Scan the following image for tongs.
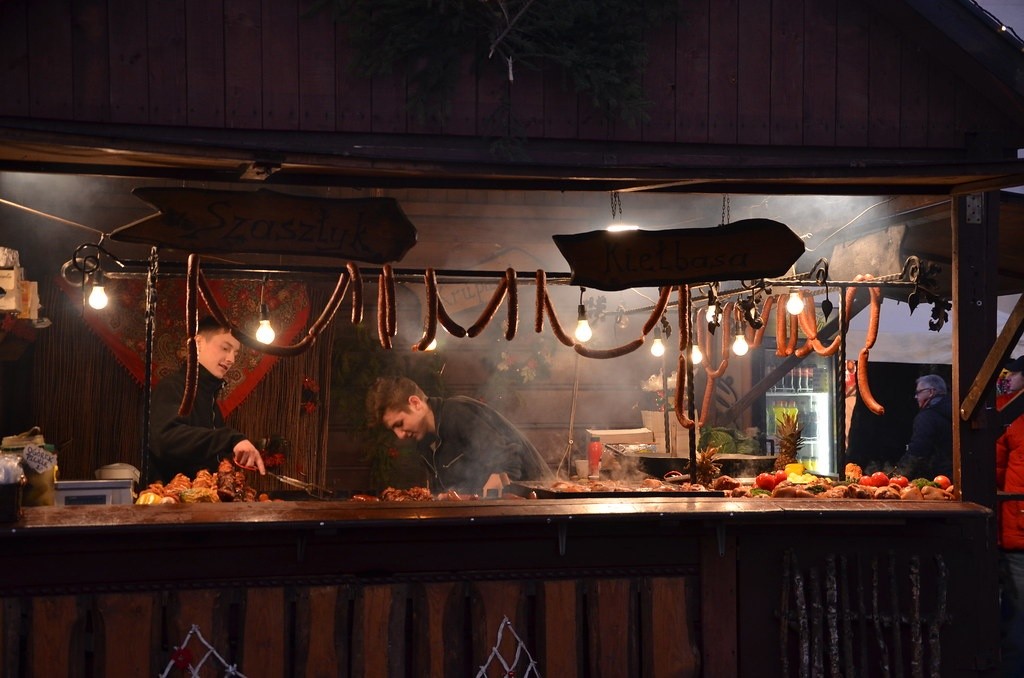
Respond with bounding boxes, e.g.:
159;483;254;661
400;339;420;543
233;455;333;501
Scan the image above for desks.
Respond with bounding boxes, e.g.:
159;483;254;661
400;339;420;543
1;497;994;678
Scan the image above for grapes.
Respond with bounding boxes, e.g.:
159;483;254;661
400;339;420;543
693;444;722;489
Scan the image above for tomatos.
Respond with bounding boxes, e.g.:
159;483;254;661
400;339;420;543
752;470;787;492
859;472;951;490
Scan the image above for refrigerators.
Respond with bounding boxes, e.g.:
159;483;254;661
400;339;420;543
734;336;845;482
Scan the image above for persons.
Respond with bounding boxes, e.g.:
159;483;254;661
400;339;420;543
146;315;266;490
993;357;1024;649
899;373;953;478
365;374;556;496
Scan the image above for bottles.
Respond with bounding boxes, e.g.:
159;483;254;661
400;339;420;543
768;359;817;393
588;437;602;476
766;400;817;437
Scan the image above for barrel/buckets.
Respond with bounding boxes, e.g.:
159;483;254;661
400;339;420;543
2;434;46;460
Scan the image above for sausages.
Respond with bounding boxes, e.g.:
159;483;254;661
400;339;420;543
178;254;883;428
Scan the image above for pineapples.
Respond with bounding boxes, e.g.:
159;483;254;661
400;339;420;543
775;413;806;471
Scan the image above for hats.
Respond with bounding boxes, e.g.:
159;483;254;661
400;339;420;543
1005;355;1024;371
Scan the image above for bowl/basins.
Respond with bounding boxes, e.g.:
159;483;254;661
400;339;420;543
575;460;601;479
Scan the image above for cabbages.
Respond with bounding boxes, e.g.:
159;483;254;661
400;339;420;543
696;427;761;455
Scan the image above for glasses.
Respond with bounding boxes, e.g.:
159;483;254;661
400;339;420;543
915;388;930;396
